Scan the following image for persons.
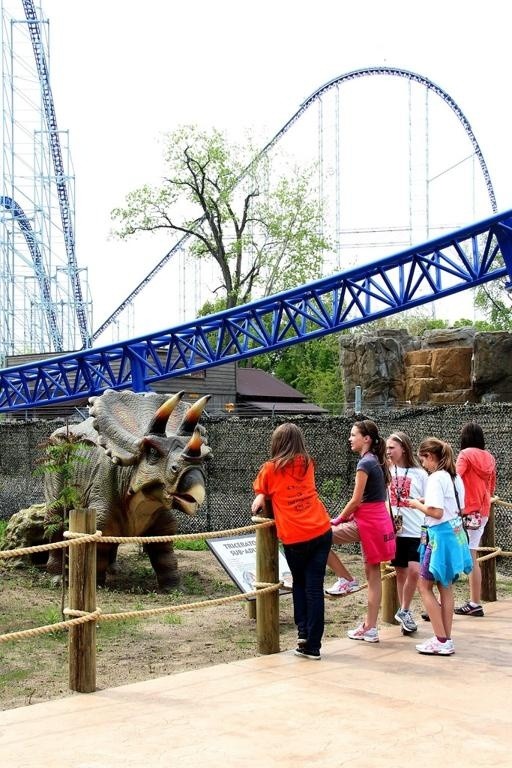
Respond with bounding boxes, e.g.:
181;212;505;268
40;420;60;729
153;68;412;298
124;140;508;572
253;424;333;660
456;423;497;618
404;436;474;656
325;420;395;643
386;433;428;635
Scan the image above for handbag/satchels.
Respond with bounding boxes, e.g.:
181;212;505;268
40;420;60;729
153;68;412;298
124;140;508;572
462;512;481;531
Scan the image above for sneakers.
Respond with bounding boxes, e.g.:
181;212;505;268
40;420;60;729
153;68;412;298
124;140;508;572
395;607;417;632
453;602;485;616
298;635;307;645
447;638;456;654
348;623;379;643
326;577;359;594
423;611;429;620
416;636;452;655
400;622;408;636
295;647;321;659
